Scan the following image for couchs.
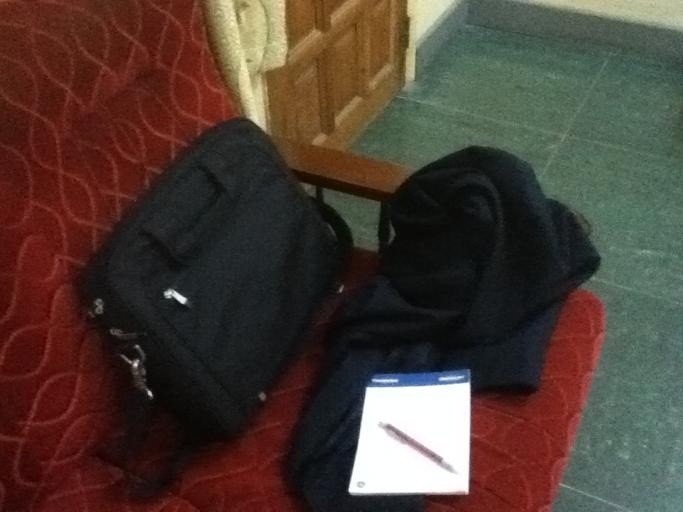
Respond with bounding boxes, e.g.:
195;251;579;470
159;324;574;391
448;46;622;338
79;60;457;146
0;0;604;512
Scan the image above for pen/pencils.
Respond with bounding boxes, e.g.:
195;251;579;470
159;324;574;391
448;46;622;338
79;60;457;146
378;420;457;474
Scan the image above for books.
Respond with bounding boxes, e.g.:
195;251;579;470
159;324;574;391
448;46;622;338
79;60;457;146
348;369;470;496
